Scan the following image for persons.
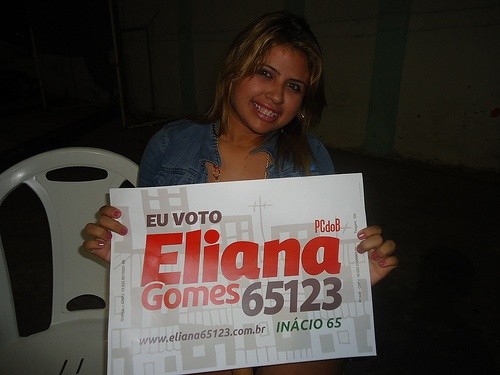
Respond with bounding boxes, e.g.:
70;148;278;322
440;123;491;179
81;11;400;375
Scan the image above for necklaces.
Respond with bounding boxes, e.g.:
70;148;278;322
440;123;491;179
211;134;269;179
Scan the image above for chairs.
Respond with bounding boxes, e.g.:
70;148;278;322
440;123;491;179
0;148;145;375
